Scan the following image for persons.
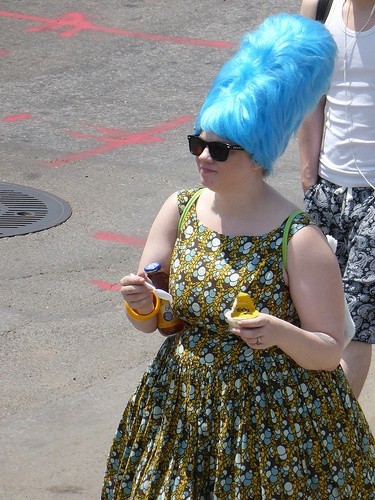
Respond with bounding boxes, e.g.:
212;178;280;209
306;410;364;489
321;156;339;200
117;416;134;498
100;13;375;500
297;0;375;399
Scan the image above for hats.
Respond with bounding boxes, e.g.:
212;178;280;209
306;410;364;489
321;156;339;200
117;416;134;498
195;13;337;176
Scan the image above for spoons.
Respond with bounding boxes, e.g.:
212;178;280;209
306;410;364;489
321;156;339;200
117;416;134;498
130;274;172;300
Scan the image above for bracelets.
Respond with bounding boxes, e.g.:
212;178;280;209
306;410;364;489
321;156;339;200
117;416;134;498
123;290;160;322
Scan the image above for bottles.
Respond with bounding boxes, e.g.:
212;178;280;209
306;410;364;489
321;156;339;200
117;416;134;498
144;262;185;336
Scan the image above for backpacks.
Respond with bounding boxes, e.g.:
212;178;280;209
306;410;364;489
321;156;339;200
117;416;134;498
178;188;356;351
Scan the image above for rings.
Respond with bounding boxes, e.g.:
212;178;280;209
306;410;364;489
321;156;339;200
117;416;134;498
257;337;261;344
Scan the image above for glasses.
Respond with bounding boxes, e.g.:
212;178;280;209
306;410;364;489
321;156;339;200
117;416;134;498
187;134;244;162
